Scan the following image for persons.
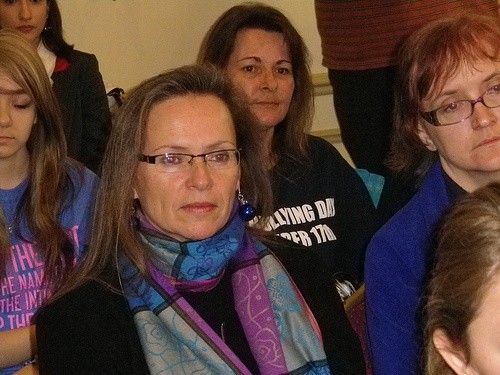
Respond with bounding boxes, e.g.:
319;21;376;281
0;0;113;179
0;26;101;375
196;1;385;301
364;12;500;375
315;0;500;221
35;64;367;375
423;181;500;375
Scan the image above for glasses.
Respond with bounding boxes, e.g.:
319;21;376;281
419;82;500;126
140;148;242;173
